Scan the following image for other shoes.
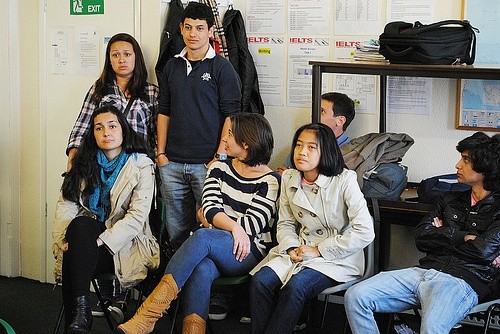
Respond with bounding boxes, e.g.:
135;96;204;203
107;302;127;323
240;295;251;325
208;292;229;320
91;298;111;316
293;303;314;331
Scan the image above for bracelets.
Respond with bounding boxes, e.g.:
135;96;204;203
156;152;166;158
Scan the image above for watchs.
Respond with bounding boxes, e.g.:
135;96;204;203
214;153;227;159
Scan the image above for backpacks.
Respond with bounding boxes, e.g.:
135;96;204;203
417;174;472;205
378;21;480;66
362;162;409;201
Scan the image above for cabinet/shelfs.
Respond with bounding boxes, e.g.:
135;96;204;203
309;58;500;271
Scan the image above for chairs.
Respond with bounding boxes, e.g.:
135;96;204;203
53;197;167;334
306;241;374;334
168;275;252;334
385;297;500;334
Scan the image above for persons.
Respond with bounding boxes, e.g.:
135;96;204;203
344;130;500;334
49;107;161;334
274;92;365;195
64;33;162;179
118;113;281;334
248;123;376;334
155;2;241;258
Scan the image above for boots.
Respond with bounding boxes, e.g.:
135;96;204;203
68;295;93;334
117;274;178;334
182;313;206;334
